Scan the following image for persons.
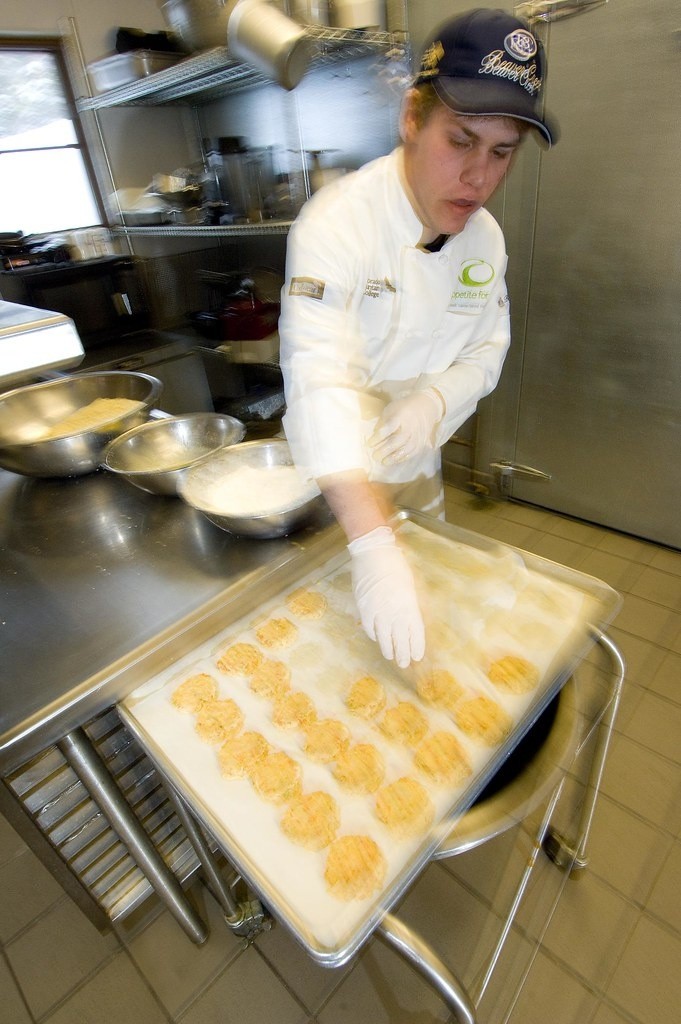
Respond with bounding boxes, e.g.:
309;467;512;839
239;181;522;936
278;8;561;670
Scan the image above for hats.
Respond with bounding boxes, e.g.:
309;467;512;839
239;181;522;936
413;7;557;150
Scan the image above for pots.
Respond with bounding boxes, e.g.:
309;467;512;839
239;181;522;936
184;279;279;341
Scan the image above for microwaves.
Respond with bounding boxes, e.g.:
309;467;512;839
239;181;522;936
1;254;159;353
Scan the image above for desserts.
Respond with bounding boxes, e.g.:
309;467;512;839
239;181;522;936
171;591;539;901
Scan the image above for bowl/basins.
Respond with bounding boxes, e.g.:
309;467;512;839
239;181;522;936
100;411;247;497
0;371;163;477
83;1;242;94
175;436;323;539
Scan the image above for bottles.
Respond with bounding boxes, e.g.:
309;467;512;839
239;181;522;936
196;132;311;223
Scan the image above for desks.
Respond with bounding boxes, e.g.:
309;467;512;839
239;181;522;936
0;408;343;952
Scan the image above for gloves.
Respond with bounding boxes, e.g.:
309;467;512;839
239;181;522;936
345;525;427;669
370;386;444;466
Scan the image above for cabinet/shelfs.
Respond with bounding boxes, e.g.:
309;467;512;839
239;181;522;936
57;0;413;376
